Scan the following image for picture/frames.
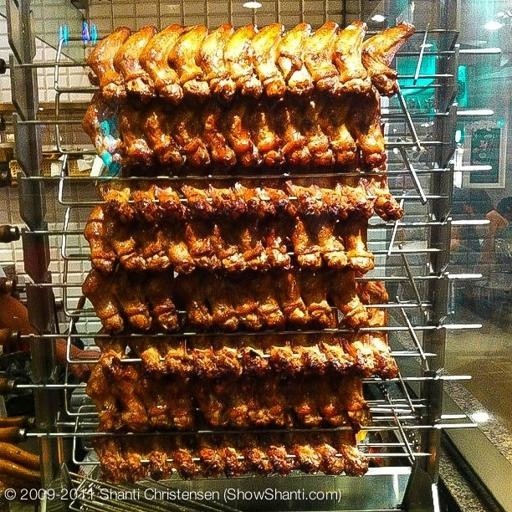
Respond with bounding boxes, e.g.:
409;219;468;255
460;121;507;189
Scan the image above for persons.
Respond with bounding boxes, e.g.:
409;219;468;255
2;271;101;381
451;185;511;279
